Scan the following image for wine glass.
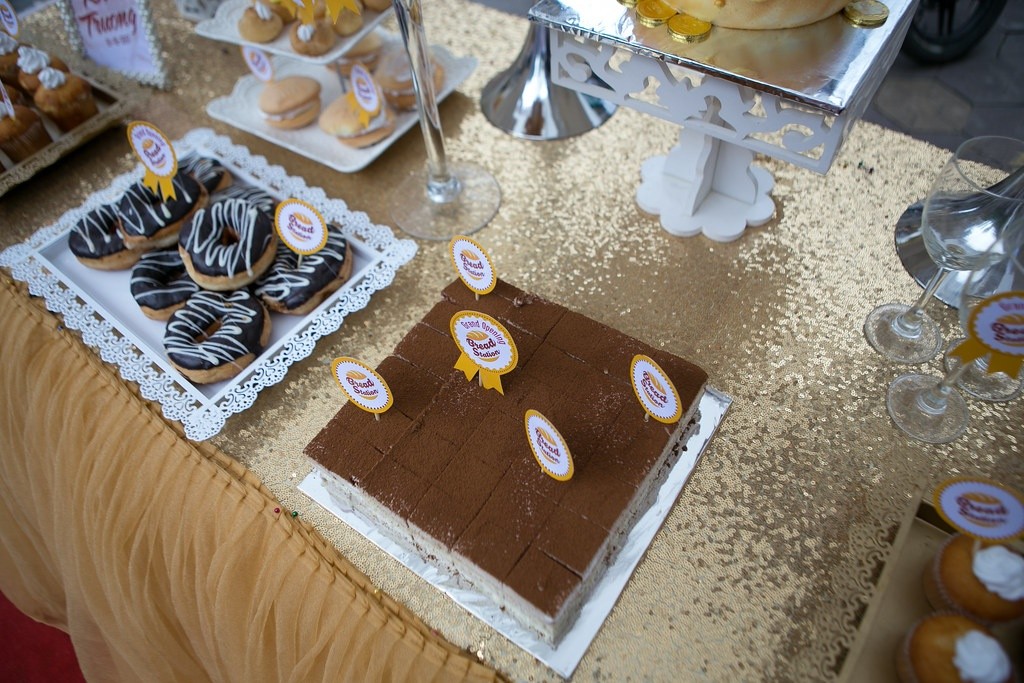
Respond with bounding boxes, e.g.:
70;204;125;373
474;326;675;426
886;218;1022;445
945;202;1023;400
390;1;502;242
866;136;1024;365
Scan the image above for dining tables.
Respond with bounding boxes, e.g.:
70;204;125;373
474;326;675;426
0;0;1024;682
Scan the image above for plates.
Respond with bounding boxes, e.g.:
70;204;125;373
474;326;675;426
1;68;134;205
0;125;418;446
204;32;477;175
193;1;395;65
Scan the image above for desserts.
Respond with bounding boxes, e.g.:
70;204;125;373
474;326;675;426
0;1;714;651
897;529;1024;682
